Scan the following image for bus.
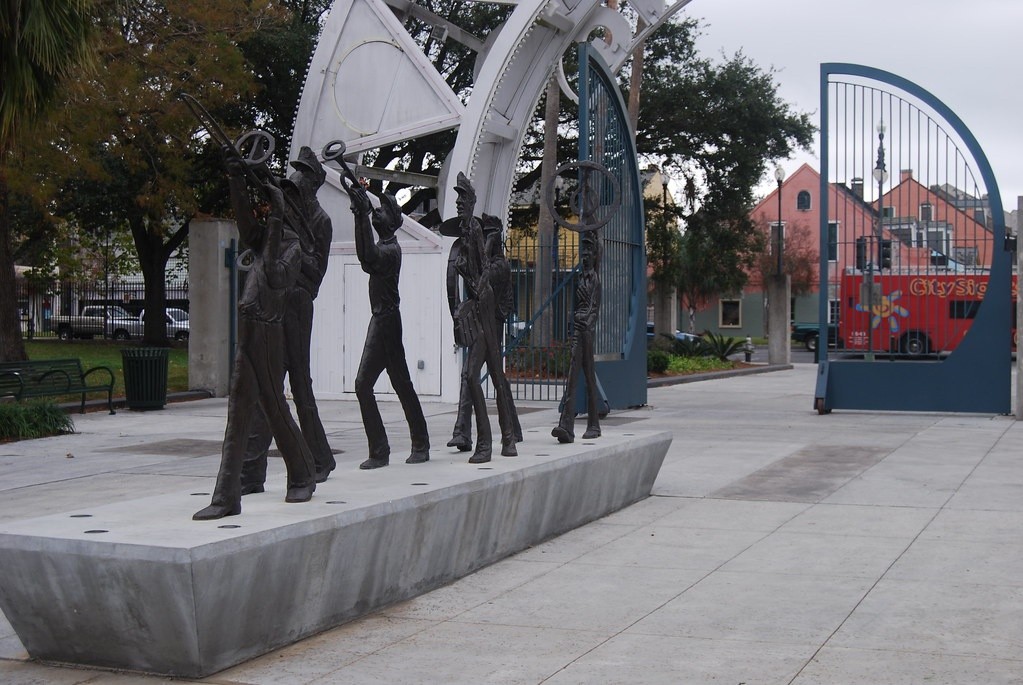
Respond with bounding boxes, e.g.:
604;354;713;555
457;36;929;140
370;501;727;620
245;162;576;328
839;266;1021;360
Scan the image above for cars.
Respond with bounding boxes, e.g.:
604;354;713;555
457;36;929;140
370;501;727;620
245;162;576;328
647;322;701;344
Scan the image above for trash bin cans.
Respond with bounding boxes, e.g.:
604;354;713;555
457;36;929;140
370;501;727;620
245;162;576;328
120;349;167;412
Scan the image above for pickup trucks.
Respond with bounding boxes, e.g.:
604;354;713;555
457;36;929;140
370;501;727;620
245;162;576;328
51;305;129;340
106;309;189;342
791;321;844;351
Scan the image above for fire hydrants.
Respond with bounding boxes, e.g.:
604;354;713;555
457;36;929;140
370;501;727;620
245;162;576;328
744;335;754;361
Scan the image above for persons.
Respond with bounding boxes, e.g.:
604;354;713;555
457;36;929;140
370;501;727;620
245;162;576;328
552;232;602;442
350;184;431;469
446;174;523;464
193;148;336;520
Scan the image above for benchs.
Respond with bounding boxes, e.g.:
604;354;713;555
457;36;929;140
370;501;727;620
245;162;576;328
0;357;117;414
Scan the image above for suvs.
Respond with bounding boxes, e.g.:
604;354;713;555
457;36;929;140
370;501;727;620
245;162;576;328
505;314;530;342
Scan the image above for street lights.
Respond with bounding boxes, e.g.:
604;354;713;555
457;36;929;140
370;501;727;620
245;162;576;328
874;120;888;267
775;164;784;274
